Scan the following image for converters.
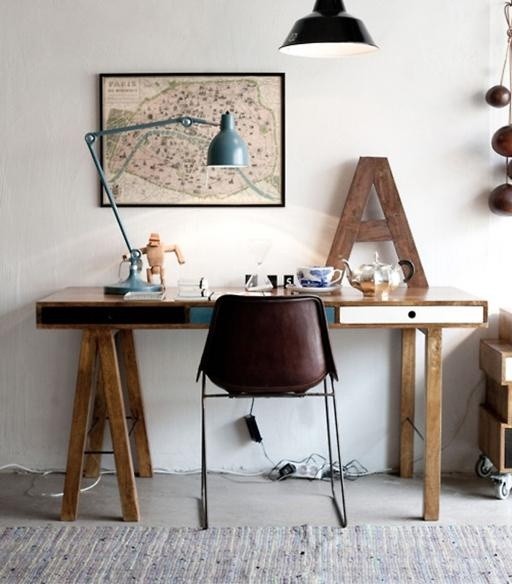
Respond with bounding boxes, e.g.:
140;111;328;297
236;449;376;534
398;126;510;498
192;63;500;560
243;410;262;446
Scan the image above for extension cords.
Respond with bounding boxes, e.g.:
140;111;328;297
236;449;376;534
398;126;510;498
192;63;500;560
282;462;323;480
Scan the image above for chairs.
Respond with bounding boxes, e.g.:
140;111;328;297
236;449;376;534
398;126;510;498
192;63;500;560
196;294;348;527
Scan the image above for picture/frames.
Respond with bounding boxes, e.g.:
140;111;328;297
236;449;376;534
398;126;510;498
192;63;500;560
97;73;285;208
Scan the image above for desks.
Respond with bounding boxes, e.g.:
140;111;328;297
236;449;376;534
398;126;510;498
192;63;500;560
37;285;488;522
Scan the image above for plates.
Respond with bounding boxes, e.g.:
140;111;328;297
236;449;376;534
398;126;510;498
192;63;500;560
285;284;342;294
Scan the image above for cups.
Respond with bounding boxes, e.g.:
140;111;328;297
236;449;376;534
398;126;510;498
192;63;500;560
297;266;344;287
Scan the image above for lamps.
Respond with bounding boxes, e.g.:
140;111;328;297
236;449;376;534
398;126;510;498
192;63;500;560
84;110;250;297
279;0;381;59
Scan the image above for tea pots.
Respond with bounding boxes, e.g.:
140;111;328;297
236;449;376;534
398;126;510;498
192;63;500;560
339;251;416;298
